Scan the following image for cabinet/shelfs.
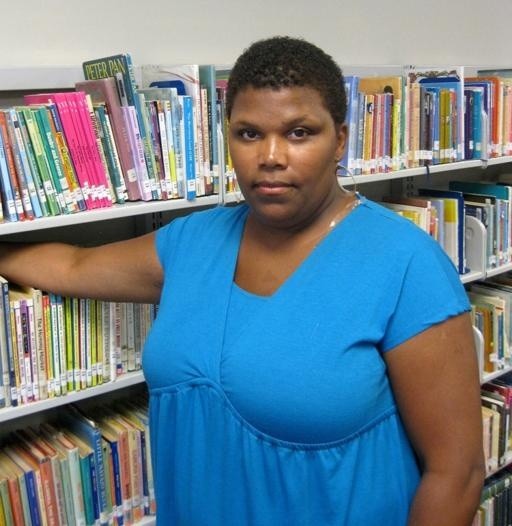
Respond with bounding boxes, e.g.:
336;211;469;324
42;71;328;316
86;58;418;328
0;153;512;525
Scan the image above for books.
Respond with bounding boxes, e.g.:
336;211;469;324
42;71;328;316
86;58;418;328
0;51;242;526
336;64;512;526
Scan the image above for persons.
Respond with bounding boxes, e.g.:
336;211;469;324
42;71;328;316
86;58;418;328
0;36;485;526
